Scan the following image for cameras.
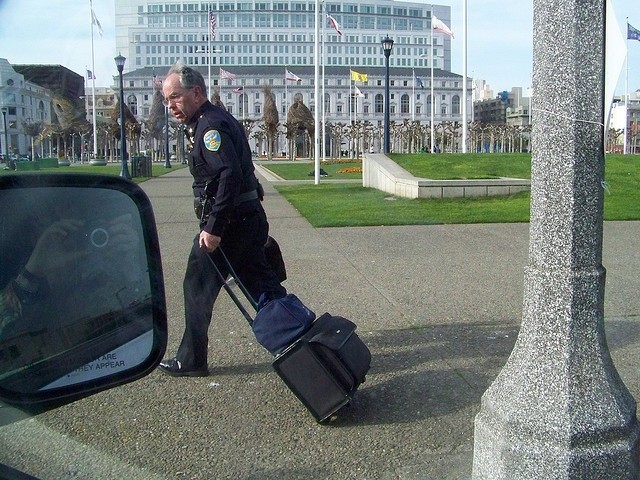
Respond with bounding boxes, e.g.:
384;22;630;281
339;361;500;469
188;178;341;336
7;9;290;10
77;212;123;259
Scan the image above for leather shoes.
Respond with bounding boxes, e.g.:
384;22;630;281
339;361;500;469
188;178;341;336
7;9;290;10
158;357;209;377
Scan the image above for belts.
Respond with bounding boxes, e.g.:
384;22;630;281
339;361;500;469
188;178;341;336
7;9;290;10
230;189;259;206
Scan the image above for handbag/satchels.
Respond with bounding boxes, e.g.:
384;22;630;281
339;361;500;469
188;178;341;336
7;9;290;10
252;291;316;356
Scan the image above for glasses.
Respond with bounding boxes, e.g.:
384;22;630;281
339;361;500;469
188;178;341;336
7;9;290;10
161;89;194;108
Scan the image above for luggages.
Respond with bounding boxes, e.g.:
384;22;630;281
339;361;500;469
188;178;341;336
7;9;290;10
200;239;371;424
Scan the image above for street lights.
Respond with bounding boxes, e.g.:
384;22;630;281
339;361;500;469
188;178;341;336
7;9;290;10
1;108;9;169
115;286;129;324
195;49;223;103
113;51;133;180
380;34;394;153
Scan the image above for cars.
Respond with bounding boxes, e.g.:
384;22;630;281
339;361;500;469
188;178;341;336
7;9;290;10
0;315;154;393
0;170;168;427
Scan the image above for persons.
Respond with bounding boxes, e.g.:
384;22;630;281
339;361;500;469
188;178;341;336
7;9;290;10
156;60;269;377
0;190;145;364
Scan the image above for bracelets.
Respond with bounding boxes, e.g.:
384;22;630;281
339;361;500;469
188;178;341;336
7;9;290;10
18;264;47;287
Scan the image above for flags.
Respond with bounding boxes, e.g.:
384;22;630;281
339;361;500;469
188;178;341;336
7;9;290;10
153;72;162;83
87;70;97;80
91;9;104;36
626;24;640;40
351;70;368;82
221;69;235;81
209;10;218;40
432;15;455;39
329;15;343;35
355;86;365;98
414;73;425;89
232;86;244;94
286;70;303;82
